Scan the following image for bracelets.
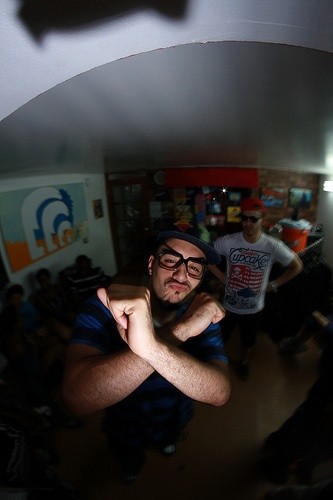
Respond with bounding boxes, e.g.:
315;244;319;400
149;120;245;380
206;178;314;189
271;281;277;293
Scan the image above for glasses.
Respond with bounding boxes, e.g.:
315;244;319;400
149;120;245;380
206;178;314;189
241;215;261;224
157;249;209;278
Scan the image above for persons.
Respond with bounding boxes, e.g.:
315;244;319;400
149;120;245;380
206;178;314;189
0;196;310;485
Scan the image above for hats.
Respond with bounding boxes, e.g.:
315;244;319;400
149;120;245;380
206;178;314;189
150;215;220;264
235;197;268;217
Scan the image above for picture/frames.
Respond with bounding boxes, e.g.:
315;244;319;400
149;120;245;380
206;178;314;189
259;186;285;209
287;187;312;209
